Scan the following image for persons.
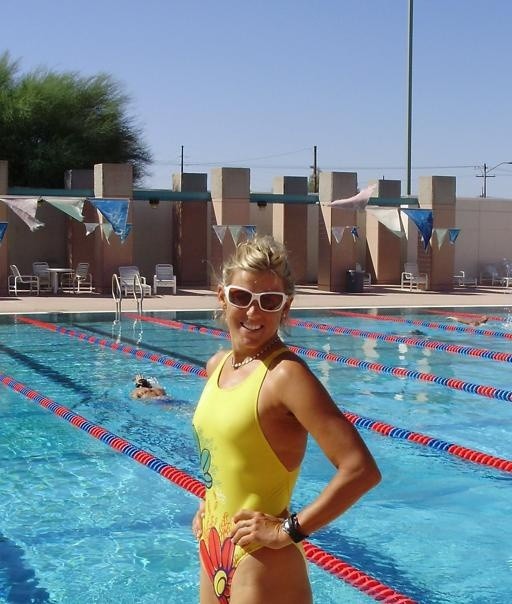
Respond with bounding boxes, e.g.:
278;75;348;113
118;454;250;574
191;227;383;603
130;370;196;415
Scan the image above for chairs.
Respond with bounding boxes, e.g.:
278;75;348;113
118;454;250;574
7;262;92;297
153;263;177;294
355;262;372;289
119;265;152;297
453;271;512;287
401;262;428;291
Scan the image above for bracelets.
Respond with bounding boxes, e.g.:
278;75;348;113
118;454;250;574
282;510;309;545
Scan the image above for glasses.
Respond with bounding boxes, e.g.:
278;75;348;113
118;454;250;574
224;285;288;312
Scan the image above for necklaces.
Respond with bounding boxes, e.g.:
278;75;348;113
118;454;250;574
230;334;281;368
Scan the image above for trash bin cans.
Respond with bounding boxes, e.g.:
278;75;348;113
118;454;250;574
347;270;364;293
48;256;66;268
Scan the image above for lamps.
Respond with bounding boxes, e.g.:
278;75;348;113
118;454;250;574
149;199;159;209
257;201;267;211
